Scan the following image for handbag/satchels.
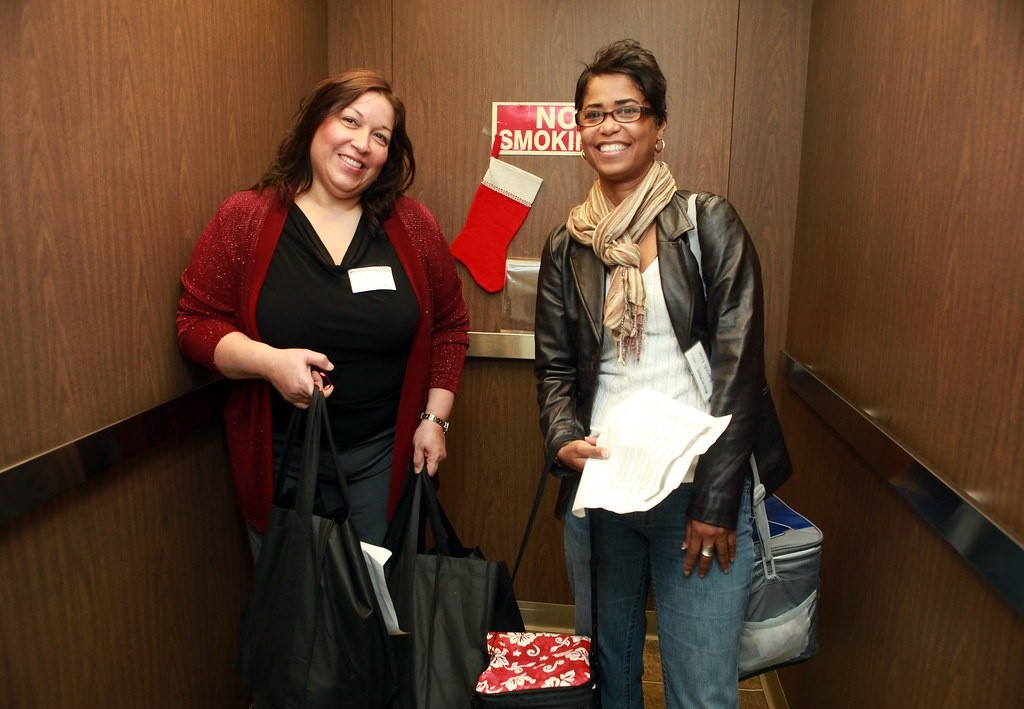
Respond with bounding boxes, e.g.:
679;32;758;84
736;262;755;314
234;385;401;709
380;457;526;709
475;628;598;709
738;492;824;681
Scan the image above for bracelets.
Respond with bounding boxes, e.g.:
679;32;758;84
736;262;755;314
419;411;451;435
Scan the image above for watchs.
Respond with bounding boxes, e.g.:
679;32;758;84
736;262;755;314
552;439;577;470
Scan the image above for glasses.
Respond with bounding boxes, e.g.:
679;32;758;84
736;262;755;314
575;104;654;128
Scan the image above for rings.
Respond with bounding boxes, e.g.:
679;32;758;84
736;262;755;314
701;545;714;558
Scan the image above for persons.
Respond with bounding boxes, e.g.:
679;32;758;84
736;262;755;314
533;37;791;709
178;69;469;708
